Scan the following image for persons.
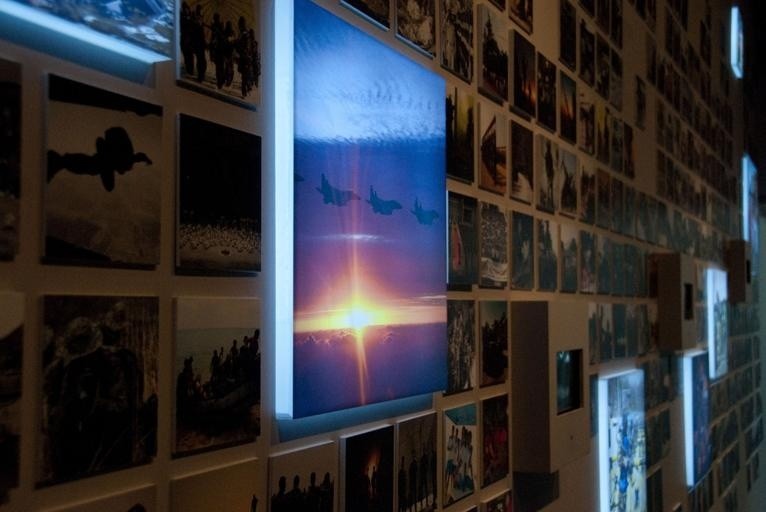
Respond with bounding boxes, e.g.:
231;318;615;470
443;425;477;497
269;469;332;511
179;327;261;417
180;4;261;99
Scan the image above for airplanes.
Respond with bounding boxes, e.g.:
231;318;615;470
311;171;441;228
